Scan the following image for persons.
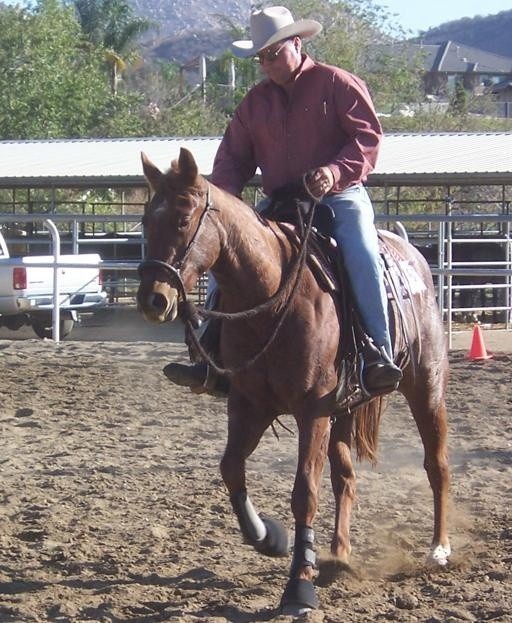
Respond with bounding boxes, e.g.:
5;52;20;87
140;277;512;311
162;6;403;398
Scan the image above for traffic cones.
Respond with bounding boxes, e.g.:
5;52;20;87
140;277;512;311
464;323;495;359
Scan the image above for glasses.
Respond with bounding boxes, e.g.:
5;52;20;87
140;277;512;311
251;38;293;66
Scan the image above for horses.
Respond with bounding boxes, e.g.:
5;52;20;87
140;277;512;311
135;146;452;617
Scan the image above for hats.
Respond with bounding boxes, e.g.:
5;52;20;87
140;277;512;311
230;5;323;57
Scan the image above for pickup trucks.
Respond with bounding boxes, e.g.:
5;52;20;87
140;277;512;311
0;228;109;341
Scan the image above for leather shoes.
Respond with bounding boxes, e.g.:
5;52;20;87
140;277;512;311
162;362;208;395
366;364;403;391
